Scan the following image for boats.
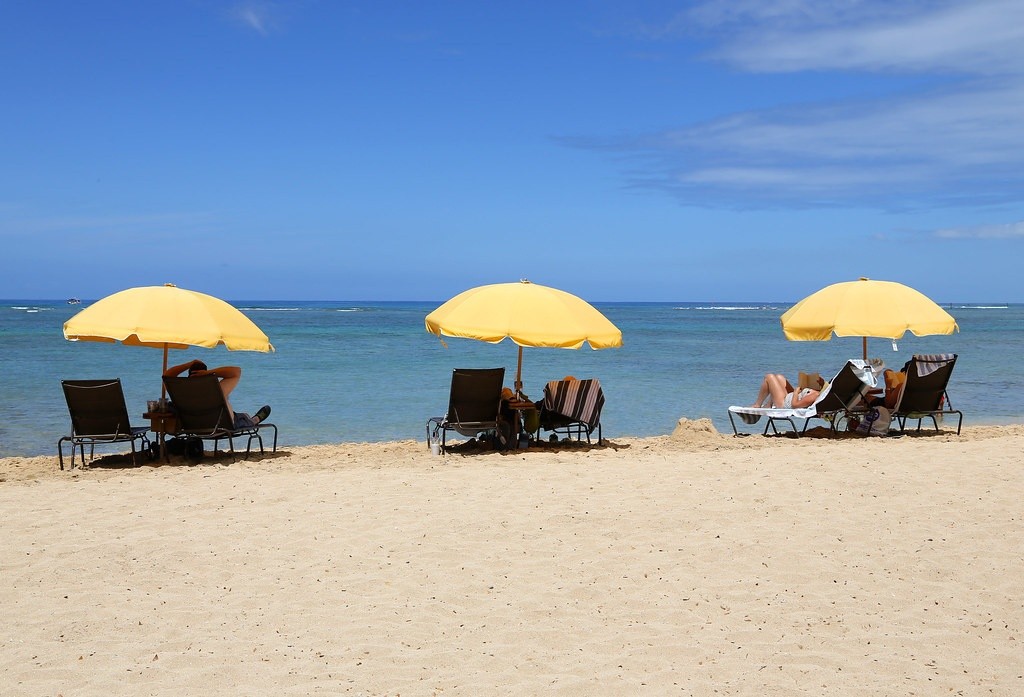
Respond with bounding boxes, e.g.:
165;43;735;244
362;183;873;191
67;299;80;305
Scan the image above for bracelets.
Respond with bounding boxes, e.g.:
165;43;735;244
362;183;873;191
885;388;891;391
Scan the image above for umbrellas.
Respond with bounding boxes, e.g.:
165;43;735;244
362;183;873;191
425;279;623;434
780;278;960;420
62;282;275;459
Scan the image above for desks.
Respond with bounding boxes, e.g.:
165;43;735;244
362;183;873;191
143;412;176;463
868;387;883;394
511;402;535;449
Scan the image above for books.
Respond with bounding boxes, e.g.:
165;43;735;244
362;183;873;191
798;372;820;391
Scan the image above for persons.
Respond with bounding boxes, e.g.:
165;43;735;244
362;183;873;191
535;375;576;410
855;360;911;408
163;358;271;430
749;374;834;408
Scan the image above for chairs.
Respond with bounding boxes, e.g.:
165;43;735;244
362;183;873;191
531;379;605;446
58;377;157;470
427;367;511;456
728;357;885;438
887;354;963;436
162;374;278;463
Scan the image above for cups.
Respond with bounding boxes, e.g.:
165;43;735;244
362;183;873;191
159;397;168;414
146;400;158;413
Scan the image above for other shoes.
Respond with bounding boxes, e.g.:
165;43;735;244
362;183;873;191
251;405;271;426
